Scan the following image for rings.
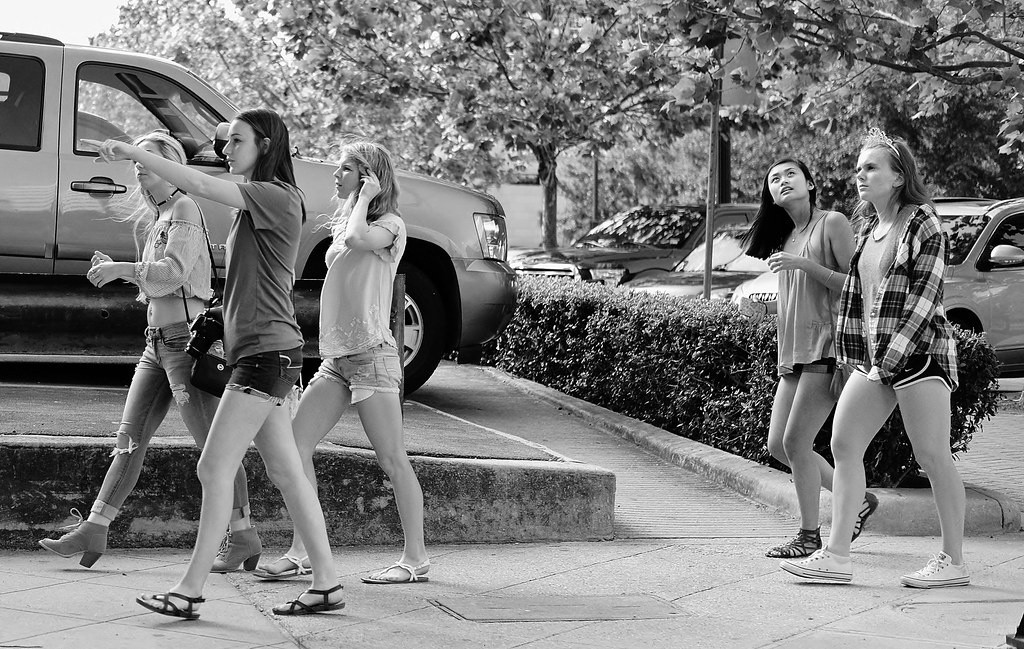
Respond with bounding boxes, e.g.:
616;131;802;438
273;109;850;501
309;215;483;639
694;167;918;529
92;274;97;279
90;268;96;273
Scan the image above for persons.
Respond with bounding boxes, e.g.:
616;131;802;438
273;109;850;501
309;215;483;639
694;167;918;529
253;143;433;585
778;127;972;588
80;109;347;620
40;131;262;573
737;157;878;559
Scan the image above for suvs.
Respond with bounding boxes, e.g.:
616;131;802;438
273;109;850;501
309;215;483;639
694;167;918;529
0;31;519;399
731;197;1024;408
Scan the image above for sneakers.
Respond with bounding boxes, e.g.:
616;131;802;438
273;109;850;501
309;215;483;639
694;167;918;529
781;546;853;582
900;551;972;588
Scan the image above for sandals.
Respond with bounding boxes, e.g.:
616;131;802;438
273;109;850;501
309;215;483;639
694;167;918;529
360;558;429;584
851;491;878;541
254;553;312;579
135;590;205;619
765;526;822;559
271;584;345;615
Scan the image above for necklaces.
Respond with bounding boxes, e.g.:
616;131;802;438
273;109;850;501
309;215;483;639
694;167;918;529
155;187;180;207
790;208;816;242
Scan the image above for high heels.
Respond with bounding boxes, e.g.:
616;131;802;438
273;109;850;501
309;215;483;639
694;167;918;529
208;525;262;573
38;508;108;568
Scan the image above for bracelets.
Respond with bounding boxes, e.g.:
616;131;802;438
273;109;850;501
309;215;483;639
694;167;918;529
824;270;834;286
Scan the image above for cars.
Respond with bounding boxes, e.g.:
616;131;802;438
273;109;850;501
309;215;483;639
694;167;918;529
506;202;760;289
615;221;783;302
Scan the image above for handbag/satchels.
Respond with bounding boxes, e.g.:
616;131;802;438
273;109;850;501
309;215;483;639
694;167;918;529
187;353;230;398
185;303;225;359
286;384;303;420
